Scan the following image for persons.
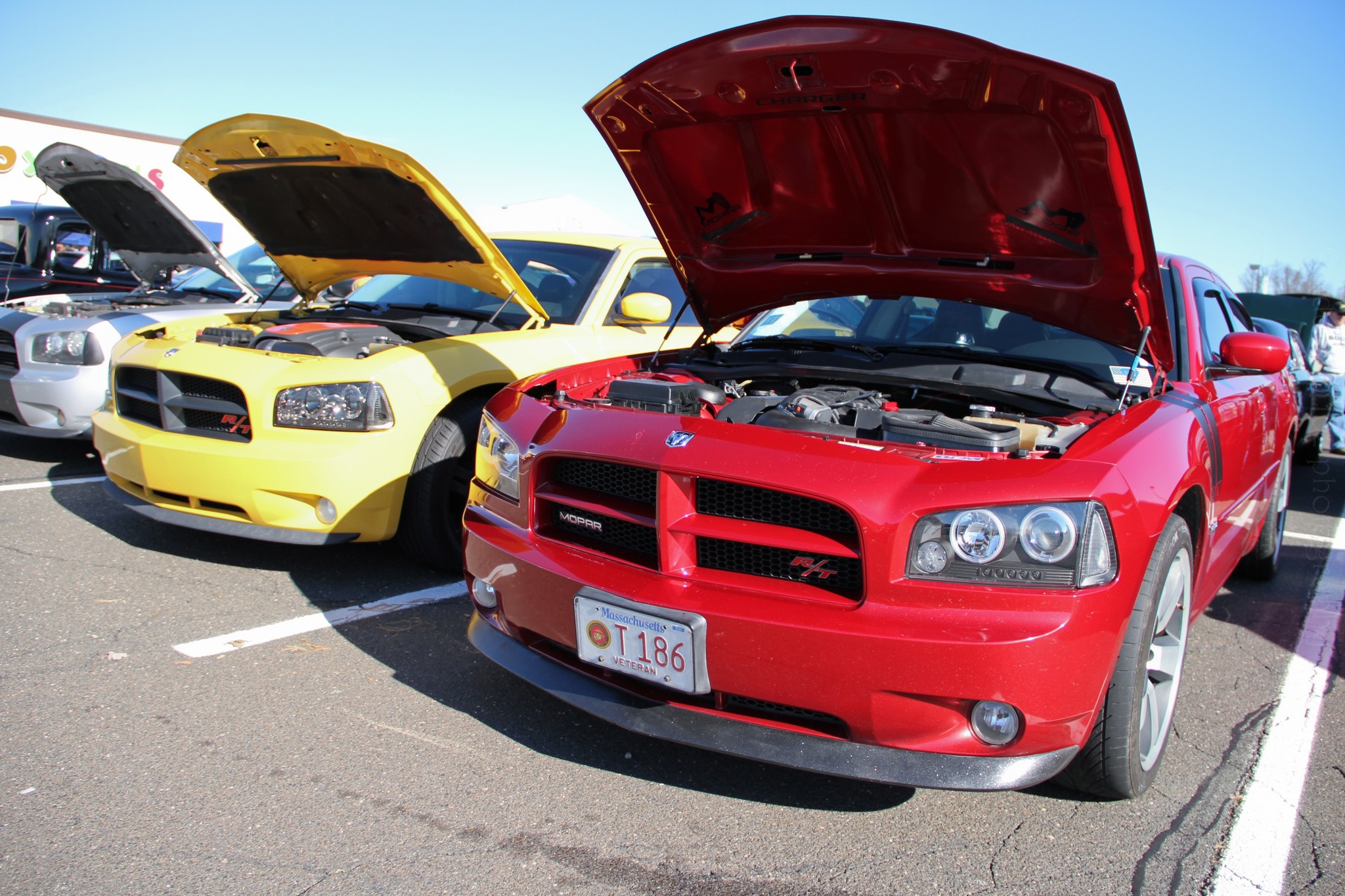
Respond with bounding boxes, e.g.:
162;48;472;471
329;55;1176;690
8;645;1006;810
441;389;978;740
1307;302;1345;455
51;243;89;259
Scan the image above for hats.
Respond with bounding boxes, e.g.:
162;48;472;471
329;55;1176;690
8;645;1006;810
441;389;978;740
1331;301;1345;313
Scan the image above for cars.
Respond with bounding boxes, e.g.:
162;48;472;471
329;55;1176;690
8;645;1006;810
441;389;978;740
1247;315;1334;470
458;13;1296;801
1;204;142;300
92;110;851;571
0;140;376;439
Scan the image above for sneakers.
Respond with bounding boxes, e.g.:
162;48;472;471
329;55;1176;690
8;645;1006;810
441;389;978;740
1330;447;1345;455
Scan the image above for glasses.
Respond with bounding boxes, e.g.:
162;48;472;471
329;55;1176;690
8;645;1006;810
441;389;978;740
1337;312;1345;316
